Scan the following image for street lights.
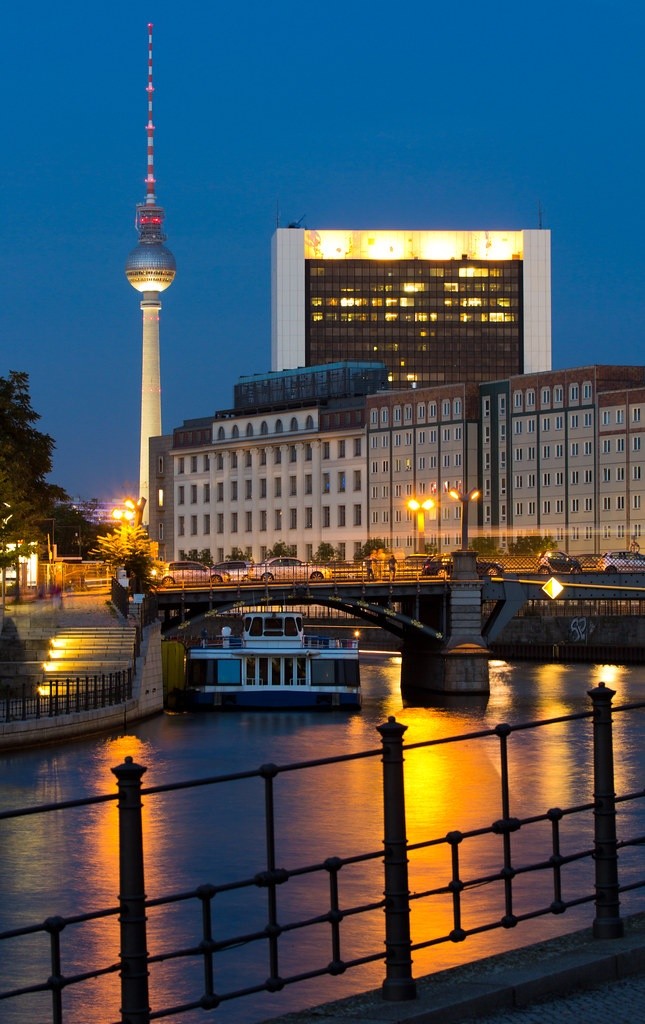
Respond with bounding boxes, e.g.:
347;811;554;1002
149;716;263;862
449;487;478;549
110;499;141;528
408;499;435;553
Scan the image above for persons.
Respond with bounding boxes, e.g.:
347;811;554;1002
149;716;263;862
388;555;399;581
365;554;379;581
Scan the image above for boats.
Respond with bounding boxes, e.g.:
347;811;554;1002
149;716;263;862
185;612;360;710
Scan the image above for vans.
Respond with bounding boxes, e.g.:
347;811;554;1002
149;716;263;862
211;561;250;581
160;561;229;584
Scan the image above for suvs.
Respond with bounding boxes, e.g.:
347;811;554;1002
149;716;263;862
423;555;503;575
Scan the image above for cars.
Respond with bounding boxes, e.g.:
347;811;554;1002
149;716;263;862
596;550;645;573
248;557;332;580
535;550;582;575
